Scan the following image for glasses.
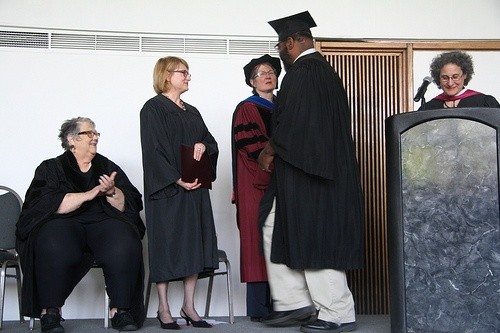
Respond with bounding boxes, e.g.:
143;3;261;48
274;39;285;50
169;70;191;78
440;72;464;82
253;72;274;80
78;130;100;139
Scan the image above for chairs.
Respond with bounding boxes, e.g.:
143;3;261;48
145;249;235;325
0;184;23;330
27;261;110;331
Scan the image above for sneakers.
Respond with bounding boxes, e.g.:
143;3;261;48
111;308;138;331
40;307;65;333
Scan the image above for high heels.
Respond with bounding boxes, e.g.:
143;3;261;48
156;310;181;329
180;308;212;328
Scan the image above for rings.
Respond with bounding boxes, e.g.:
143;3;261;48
198;148;201;150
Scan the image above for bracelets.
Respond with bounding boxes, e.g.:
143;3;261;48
106;189;115;197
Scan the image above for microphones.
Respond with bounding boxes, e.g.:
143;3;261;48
414;76;433;102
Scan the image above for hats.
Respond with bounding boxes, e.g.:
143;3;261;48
243;53;282;89
267;10;317;40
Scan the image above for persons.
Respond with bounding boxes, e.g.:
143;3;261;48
15;116;147;333
257;11;366;333
419;52;500;111
232;55;282;322
140;57;220;330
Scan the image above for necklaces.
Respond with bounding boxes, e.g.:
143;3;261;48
162;93;186;111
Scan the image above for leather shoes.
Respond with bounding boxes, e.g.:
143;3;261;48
300;318;356;332
264;306;315;324
